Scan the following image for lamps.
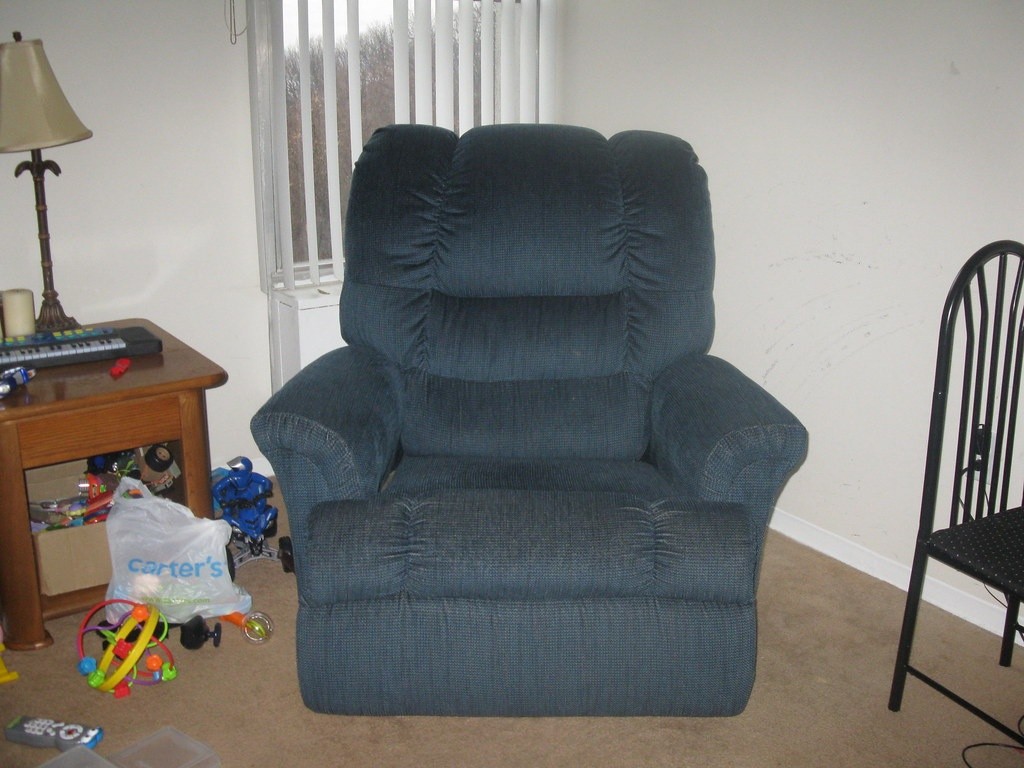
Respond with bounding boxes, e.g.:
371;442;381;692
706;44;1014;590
0;31;93;332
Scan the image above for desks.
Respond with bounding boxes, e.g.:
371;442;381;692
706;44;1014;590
0;316;229;652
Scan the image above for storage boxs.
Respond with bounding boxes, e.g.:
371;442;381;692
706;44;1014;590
27;469;129;611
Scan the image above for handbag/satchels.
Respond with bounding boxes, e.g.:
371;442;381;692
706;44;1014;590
105;476;251;623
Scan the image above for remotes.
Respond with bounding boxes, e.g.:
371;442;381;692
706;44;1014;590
5;716;104;751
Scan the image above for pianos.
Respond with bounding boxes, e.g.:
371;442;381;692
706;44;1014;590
0;328;161;370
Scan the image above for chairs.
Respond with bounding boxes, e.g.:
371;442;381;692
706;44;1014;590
248;123;807;717
885;237;1024;747
